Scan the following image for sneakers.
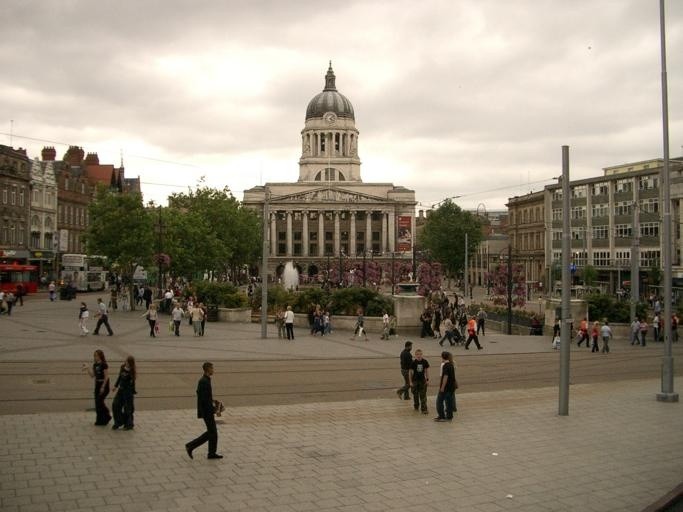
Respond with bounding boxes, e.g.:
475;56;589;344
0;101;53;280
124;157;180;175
150;331;206;339
433;407;457;420
207;452;223;459
438;340;484;351
278;336;294;340
94;415;134;431
630;341;646;347
310;330;369;342
185;442;194;460
577;343;610;354
79;331;113;337
396;389;429;414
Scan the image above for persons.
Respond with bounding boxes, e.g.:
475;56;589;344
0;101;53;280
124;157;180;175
538;288;678;354
185;362;223;459
81;349;112;426
274;282;489;422
0;281;24;315
110;355;137;430
41;275;76;302
79;278;207;338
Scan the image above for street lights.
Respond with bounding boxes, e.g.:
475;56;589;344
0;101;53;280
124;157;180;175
152;204;169;300
323;248;405;296
496;243;522;333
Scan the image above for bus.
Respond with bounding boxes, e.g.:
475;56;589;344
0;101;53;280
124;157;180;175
0;256;39;295
60;253;108;292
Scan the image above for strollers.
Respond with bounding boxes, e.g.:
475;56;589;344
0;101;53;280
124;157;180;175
450;328;466;347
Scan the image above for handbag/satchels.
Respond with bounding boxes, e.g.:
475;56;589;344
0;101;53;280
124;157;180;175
168;321;175;332
198;308;203;321
145;310;150;320
180;311;184;319
154;324;160;333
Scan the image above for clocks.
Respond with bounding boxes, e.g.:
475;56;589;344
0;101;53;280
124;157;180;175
323;111;337;126
